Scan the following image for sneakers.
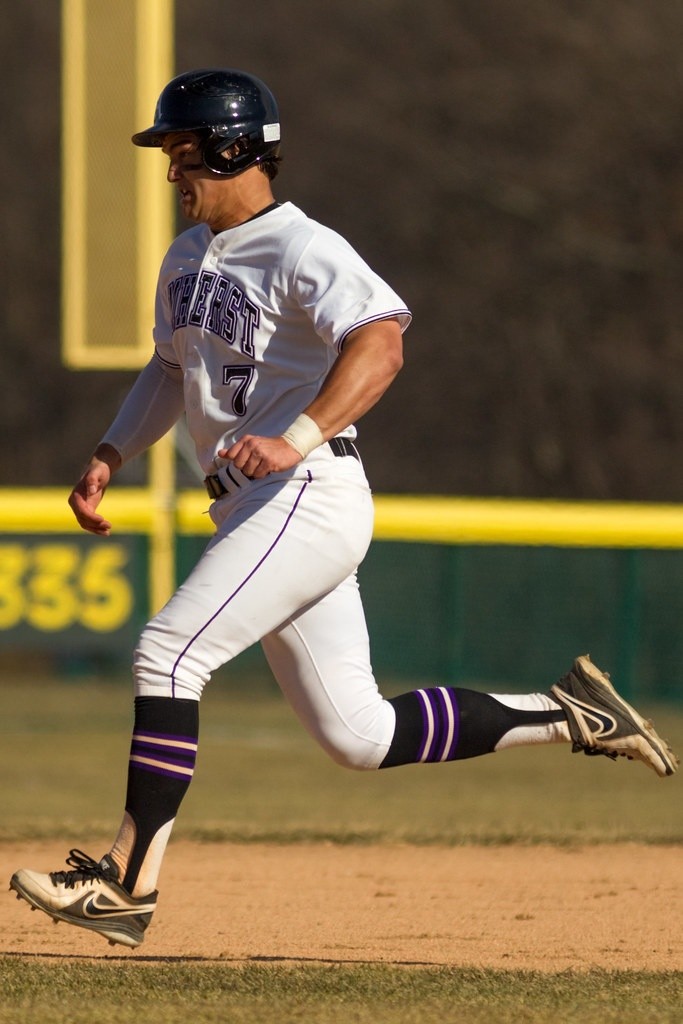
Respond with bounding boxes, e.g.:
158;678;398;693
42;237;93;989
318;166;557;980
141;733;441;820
9;848;160;948
549;655;681;778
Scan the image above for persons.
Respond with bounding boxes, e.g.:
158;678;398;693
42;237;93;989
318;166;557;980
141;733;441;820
13;70;679;947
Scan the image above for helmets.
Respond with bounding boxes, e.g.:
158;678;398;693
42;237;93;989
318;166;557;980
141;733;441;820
132;69;282;179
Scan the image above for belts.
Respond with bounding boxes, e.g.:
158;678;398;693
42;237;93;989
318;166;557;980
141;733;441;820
204;437;358;502
